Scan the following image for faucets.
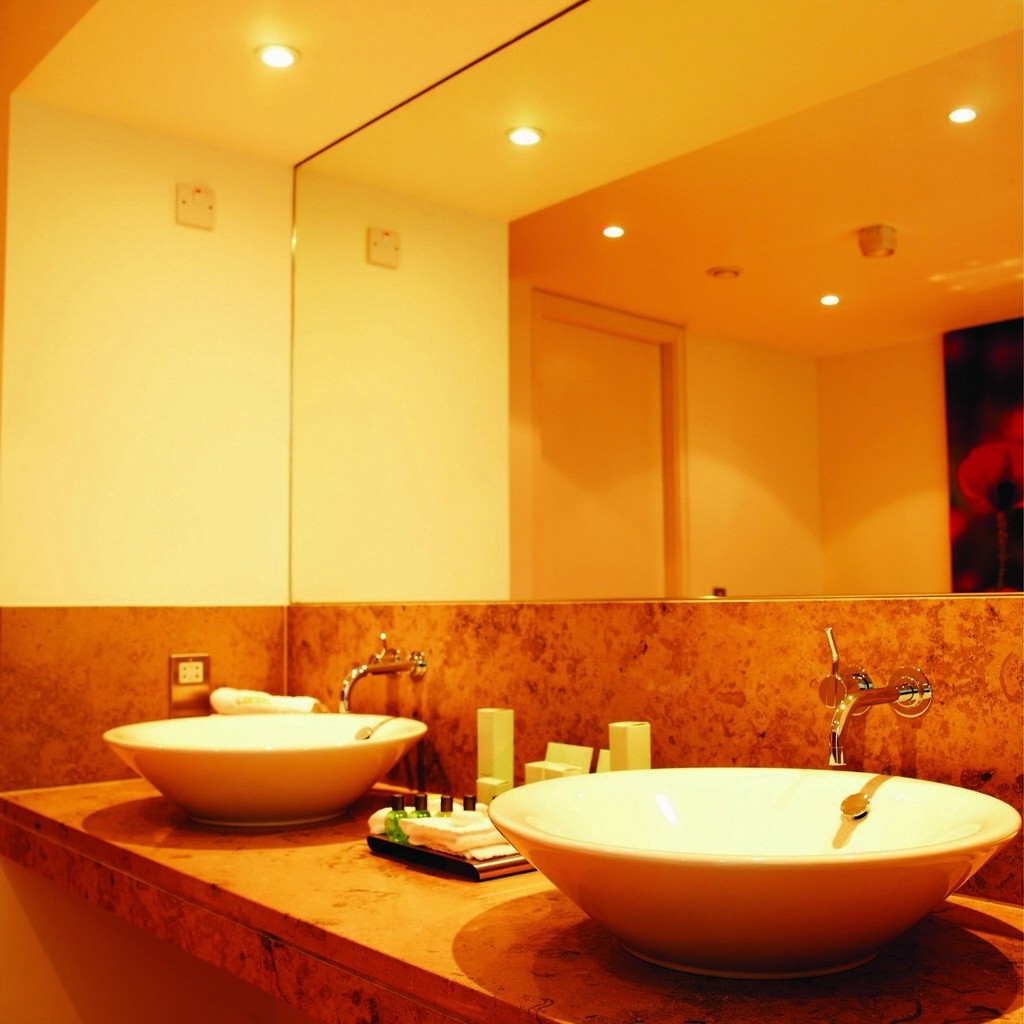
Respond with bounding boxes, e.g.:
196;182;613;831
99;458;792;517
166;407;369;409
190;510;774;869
339;638;424;703
829;666;932;765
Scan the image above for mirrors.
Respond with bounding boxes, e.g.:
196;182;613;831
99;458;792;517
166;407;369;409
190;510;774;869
288;0;1024;604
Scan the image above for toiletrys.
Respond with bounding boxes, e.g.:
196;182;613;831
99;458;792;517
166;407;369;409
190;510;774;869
368;792;478;841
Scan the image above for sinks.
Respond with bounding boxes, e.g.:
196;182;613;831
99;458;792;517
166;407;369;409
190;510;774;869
101;713;428;825
490;762;1022;973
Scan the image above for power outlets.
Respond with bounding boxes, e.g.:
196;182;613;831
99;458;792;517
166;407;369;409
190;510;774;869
170;654;211;718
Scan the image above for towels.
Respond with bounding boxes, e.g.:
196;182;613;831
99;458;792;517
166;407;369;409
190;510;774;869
399;818;516;858
210;687;324;712
